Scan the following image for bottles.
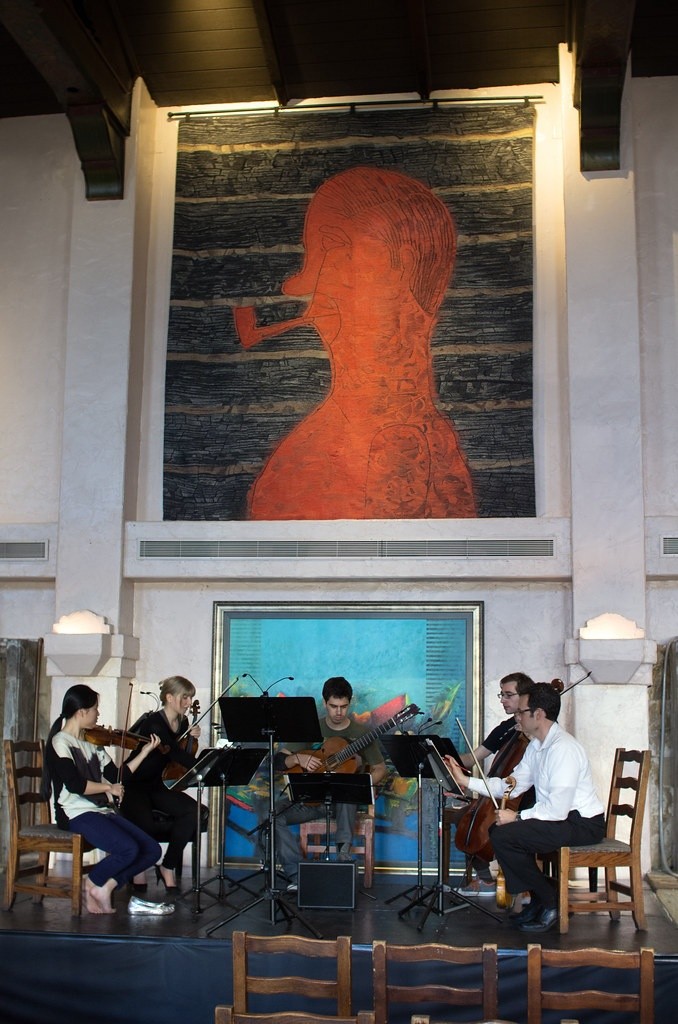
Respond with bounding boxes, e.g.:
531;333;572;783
497;866;512;908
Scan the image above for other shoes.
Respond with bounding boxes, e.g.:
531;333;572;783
127;895;175;916
336;842;351;861
287;882;298;891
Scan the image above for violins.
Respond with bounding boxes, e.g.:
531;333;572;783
161;699;201;792
495;777;517;910
84;723;170;755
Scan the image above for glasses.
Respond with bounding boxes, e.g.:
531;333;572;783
514;709;531;717
498;693;520;699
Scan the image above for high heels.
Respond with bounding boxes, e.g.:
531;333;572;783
155;865;181;894
131;877;147;893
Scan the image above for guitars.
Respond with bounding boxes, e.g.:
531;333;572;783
281;703;425;808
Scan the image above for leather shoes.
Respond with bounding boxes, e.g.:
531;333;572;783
518;908;557;930
509;903;544;923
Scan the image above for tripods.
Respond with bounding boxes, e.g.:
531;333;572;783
380;730;504;932
206;696;324;941
188;746;273;899
162;749;243;916
227;749;294;890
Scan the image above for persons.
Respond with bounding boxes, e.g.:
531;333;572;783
128;676;209;895
263;677;387;893
453;672;535;904
442;683;605;933
40;684;162;914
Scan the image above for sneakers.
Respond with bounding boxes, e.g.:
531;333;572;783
454;878;496;897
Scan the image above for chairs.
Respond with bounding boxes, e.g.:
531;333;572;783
543;747;653;934
4;738;98;920
527;944;654;1024
369;939;500;1024
300;803;376;888
233;931;353;1017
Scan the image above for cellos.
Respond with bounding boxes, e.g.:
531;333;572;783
449;677;565;904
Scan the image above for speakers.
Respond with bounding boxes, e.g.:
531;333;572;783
297;861;358;909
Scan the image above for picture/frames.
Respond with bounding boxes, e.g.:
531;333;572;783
207;600;485;875
